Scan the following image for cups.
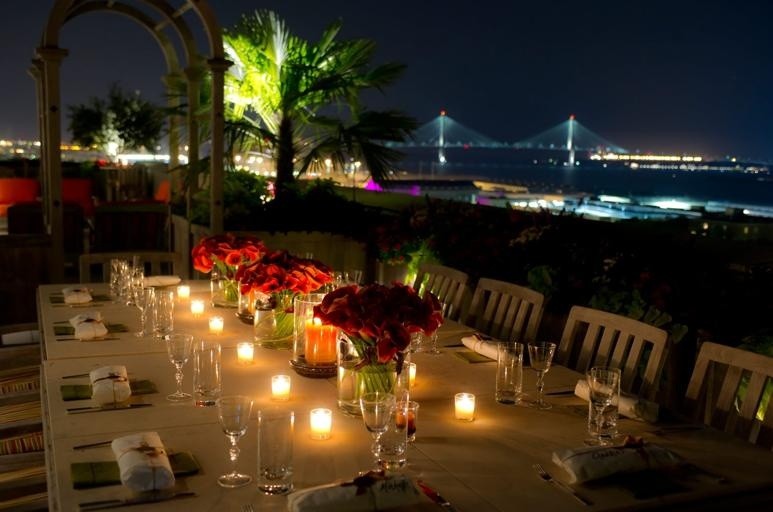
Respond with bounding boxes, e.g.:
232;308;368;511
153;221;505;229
495;341;526;405
270;374;292;403
209;315;224;336
394;362;416;388
454;390;476;422
587;365;618;439
236;342;254;365
152;290;174;338
256;405;295;494
191;300;205;320
177;285;190;302
395;401;420;443
309;407;333;441
193;340;223;407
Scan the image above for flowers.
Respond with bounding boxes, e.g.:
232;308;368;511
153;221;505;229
192;233;267;281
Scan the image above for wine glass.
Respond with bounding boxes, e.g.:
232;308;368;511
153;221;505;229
164;334;193;403
528;342;557;410
216;394;255;487
112;259;155;339
583;369;615;448
359;390;397;458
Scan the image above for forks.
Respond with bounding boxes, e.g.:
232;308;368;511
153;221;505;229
531;462;595;508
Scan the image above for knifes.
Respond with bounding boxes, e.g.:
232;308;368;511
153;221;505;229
76;491;198;511
546;389;577;395
52;303;153;414
